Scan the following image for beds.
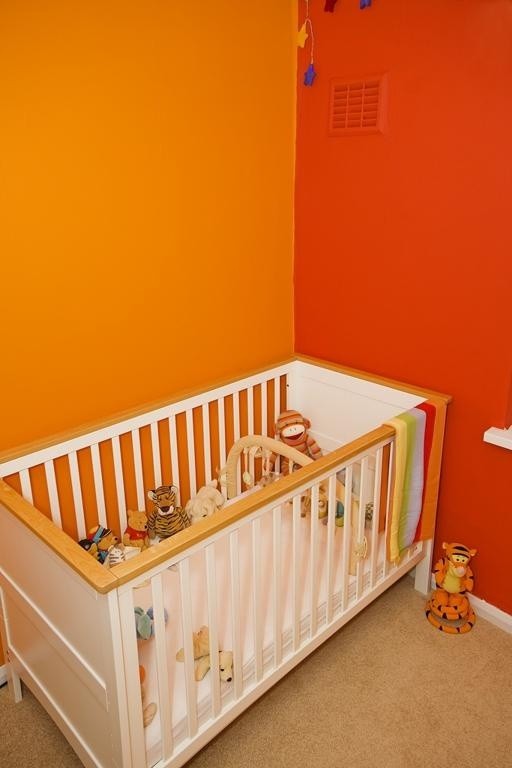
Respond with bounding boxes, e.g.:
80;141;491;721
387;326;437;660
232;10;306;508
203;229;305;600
0;348;458;768
